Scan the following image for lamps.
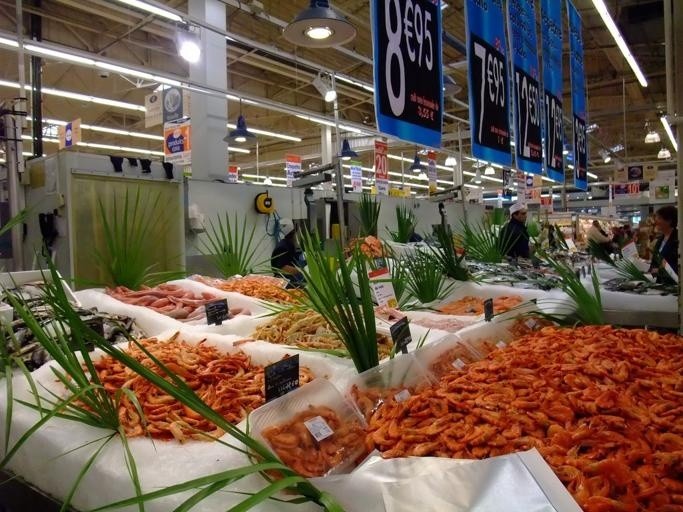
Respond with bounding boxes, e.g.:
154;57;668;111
472;161;485;185
598;148;612;164
485;161;496;176
405;144;426;173
645;120;660;143
655;146;671;159
445;147;457;166
282;0;358;48
172;20;203;64
221;98;257;146
335;130;358;161
263;150;273;185
590;0;650;90
311;72;337;102
656;110;679;153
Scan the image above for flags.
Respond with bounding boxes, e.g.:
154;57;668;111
500;167;552;215
368;0;442;151
373;140;388;195
285;154;301;188
612;164;676;206
163;118;191;165
56;118;82;149
427;151;437;195
228;166;239;182
464;0;590;191
144;86;190;128
349;161;362;193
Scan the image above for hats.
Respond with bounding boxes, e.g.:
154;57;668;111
278;217;296;237
509;201;524;216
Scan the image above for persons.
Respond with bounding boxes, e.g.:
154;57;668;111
269;227;310;294
491;201;530;259
587;217;637;268
645;205;679;287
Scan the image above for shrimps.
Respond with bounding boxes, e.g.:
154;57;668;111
0;276;683;512
